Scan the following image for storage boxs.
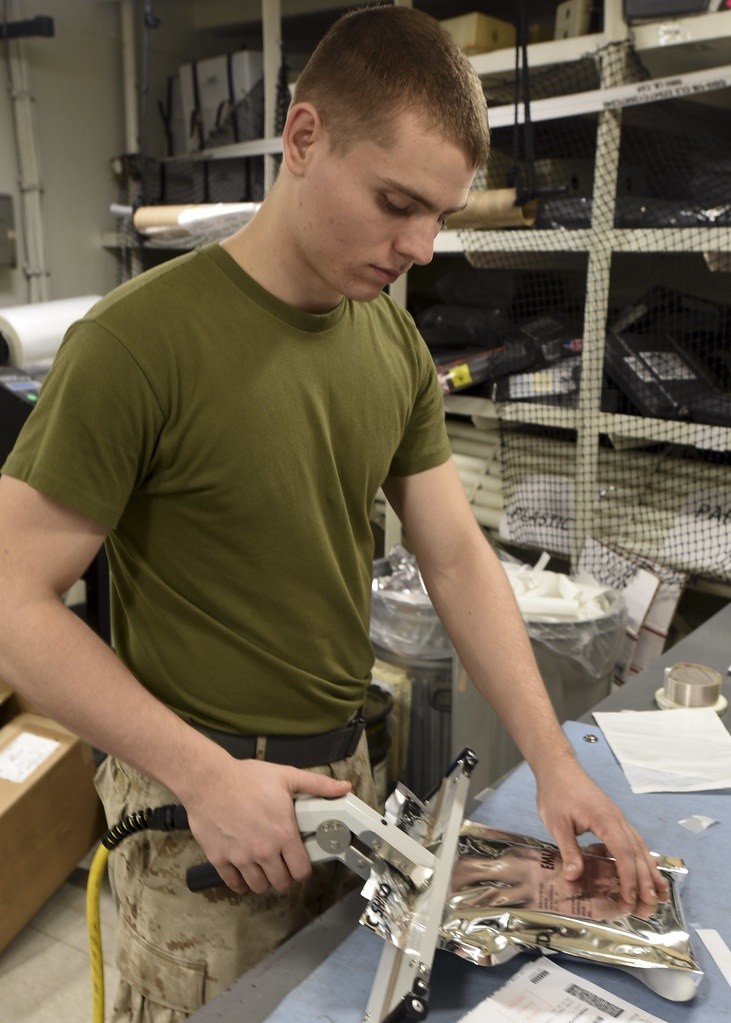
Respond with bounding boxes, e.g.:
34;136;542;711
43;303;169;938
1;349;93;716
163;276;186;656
0;716;99;959
433;14;518;59
178;47;258;147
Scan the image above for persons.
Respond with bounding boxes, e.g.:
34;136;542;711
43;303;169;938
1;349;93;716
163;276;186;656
0;5;668;1023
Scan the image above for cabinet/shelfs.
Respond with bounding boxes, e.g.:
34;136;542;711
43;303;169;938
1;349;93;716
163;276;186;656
115;0;731;599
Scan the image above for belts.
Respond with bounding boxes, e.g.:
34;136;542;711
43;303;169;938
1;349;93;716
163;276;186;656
190;719;365;769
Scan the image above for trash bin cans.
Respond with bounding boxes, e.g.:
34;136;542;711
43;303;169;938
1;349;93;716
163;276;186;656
370;554;451;805
452;567;620;802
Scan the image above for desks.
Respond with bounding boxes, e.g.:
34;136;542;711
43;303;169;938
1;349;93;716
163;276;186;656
184;606;730;1023
